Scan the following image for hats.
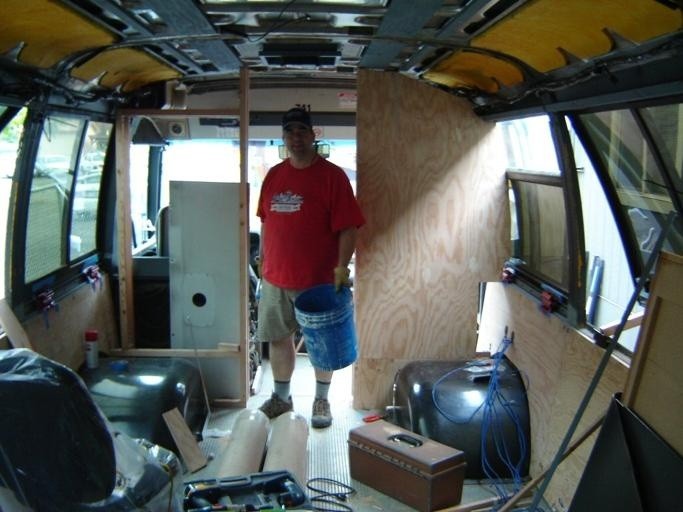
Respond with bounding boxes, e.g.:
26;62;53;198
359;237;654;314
281;108;313;131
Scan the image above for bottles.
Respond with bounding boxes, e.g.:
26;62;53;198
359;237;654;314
84;330;100;368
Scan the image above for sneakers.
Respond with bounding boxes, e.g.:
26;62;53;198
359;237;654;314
258;391;294;419
310;396;334;430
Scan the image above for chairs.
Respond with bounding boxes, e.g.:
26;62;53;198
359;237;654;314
154;205;261;352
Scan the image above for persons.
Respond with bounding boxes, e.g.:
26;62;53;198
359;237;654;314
256;108;365;427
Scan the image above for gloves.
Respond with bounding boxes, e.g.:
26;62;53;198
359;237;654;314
333;267;354;293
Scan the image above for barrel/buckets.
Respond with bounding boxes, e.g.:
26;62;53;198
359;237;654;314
293;284;357;372
